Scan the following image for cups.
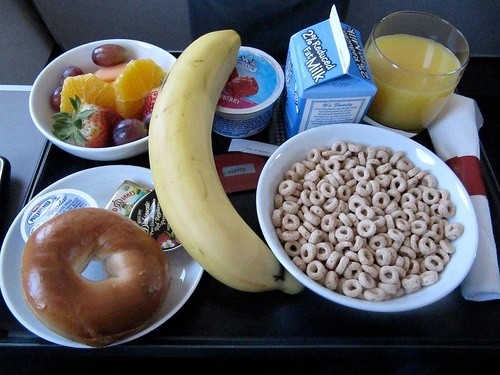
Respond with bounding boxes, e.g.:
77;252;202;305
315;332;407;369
363;10;470;138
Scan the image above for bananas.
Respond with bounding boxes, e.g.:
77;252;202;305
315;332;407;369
148;29;305;294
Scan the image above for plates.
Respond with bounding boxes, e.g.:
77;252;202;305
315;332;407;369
0;164;204;348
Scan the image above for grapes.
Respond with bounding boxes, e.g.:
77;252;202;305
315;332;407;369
112;118;147;145
60;66;84;85
92;44;126;66
51;86;64;111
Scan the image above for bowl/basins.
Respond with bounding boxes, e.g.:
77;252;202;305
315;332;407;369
213;46;283;138
256;123;479;313
28;38;178;160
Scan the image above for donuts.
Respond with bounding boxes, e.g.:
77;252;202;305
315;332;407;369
22;207;171;346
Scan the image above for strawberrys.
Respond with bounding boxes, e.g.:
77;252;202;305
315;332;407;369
50;96;125;148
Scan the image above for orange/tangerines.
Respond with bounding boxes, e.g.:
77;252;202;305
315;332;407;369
59;72;115;112
113;58;164;118
